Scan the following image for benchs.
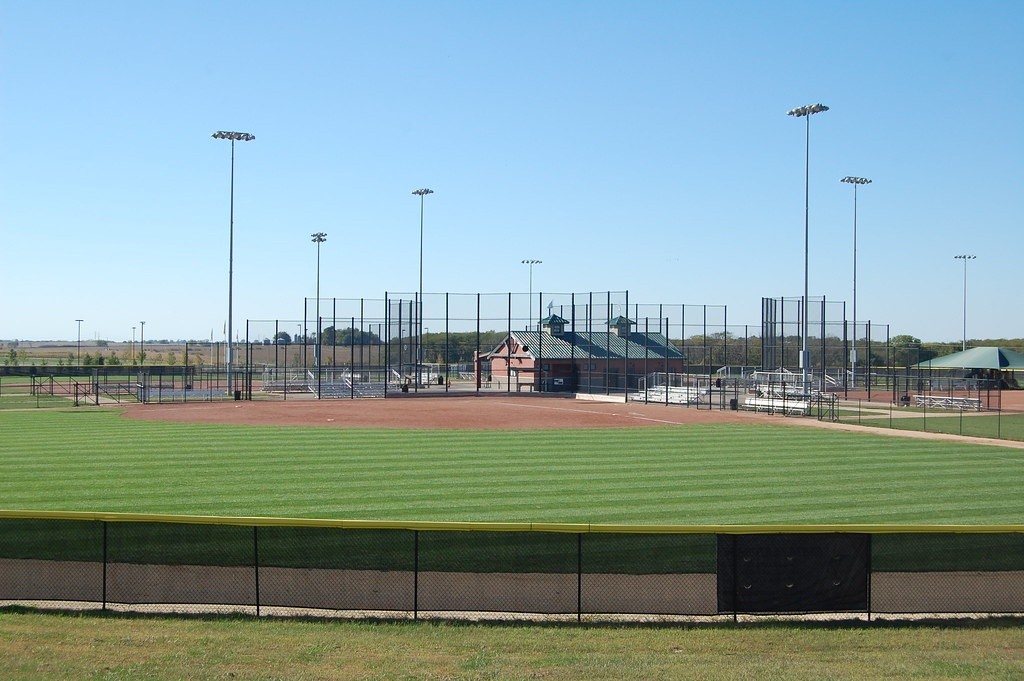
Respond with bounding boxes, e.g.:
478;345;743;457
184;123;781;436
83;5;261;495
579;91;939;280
914;395;984;411
261;378;400;398
741;397;808;416
628;385;710;405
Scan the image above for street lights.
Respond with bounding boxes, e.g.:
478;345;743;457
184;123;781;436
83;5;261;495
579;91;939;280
787;103;829;401
839;175;872;389
213;130;256;396
954;254;977;353
412;189;433;368
75;319;84;366
311;231;327;367
132;327;136;362
522;259;542;333
141;320;147;362
297;324;302;366
